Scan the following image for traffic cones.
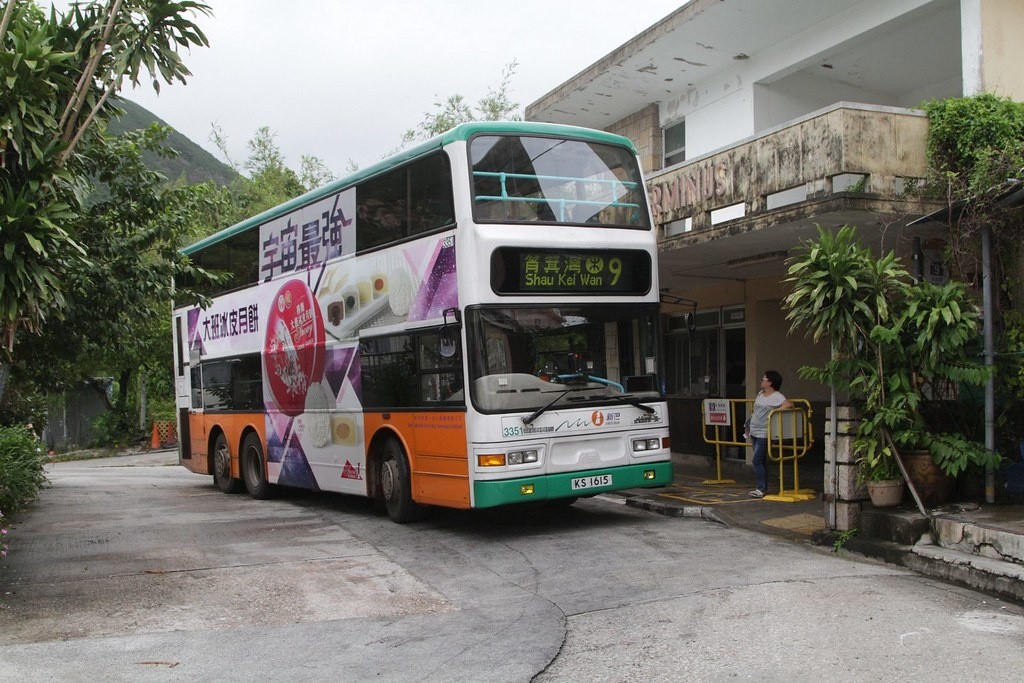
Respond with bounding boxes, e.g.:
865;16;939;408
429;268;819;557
150;421;163;450
164;421;179;448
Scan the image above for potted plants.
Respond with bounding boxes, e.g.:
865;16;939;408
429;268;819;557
781;222;1024;507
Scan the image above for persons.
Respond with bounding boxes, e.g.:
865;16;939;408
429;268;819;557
744;370;794;498
25;422;41;454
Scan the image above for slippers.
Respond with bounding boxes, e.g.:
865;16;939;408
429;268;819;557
748;489;765;498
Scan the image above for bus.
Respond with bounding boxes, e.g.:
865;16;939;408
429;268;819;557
166;121;678;525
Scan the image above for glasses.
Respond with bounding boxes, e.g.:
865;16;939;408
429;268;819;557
760;378;769;382
